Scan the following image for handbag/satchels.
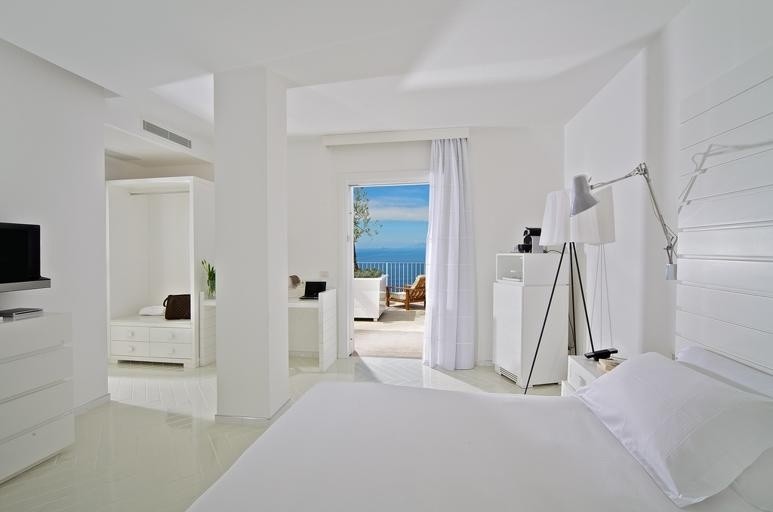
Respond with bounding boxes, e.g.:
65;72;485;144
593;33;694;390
163;294;191;320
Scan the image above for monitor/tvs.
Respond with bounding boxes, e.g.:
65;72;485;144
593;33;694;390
0;222;40;283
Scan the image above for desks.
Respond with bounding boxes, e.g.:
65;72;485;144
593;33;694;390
199;288;338;371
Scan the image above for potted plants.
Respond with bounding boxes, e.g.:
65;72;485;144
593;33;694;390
202;258;216;299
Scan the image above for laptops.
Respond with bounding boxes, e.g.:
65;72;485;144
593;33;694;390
300;282;326;299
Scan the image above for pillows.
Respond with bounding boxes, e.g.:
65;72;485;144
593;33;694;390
570;352;773;507
674;344;773;512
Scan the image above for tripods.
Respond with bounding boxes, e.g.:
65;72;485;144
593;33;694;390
524;243;595;395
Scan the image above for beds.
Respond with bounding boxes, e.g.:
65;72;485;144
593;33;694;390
185;342;773;512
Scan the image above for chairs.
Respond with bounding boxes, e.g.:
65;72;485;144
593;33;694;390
386;274;425;310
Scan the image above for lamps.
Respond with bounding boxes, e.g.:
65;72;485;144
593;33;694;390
569;162;677;281
522;189;595;394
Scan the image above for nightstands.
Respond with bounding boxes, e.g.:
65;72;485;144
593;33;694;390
561;353;632;394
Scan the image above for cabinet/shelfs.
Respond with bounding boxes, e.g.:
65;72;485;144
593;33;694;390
491;250;568;390
0;311;77;485
105;176;215;369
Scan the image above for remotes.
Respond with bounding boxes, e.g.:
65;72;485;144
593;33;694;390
583;347;618;358
